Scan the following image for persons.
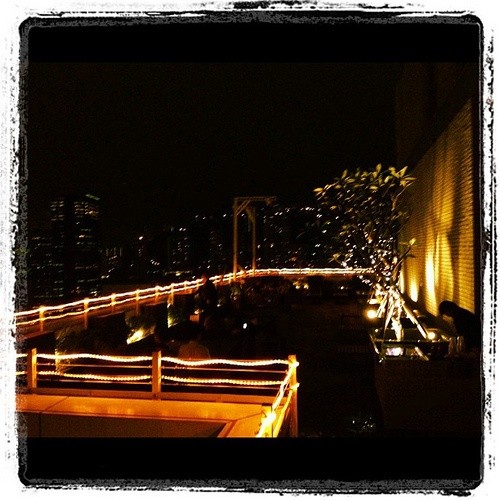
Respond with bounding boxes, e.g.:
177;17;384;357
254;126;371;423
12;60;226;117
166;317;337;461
194;273;218;314
437;301;482;359
